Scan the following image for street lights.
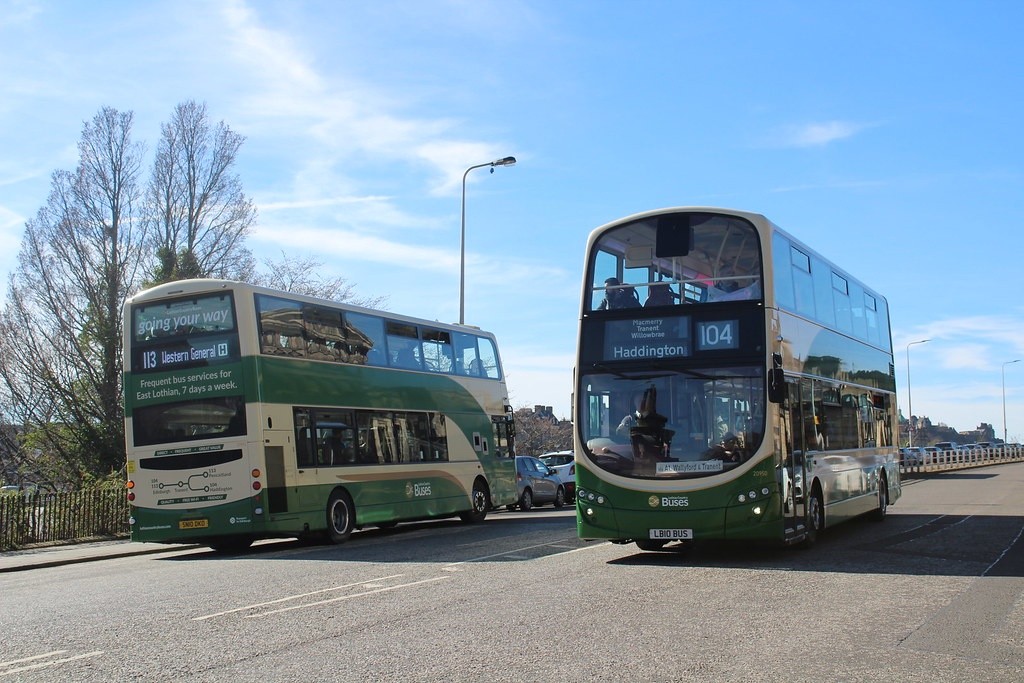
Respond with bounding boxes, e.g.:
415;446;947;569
907;339;933;446
461;157;518;323
1002;359;1020;443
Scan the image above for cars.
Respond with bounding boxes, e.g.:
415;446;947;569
964;444;987;458
906;447;932;464
958;446;972;461
996;443;1024;459
924;447;948;460
900;448;920;465
515;456;566;512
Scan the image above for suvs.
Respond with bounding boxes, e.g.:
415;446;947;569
538;450;575;504
977;442;1001;457
936;442;960;462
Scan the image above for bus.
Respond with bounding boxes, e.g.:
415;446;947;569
567;205;902;552
122;278;519;554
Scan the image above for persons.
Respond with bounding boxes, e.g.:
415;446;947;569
298;427;313;465
714;265;745;293
721;432;744;450
615;392;664;442
322;428;346;464
596;278;642;309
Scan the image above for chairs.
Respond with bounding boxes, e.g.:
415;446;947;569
669;286;675;301
620;283;640;302
